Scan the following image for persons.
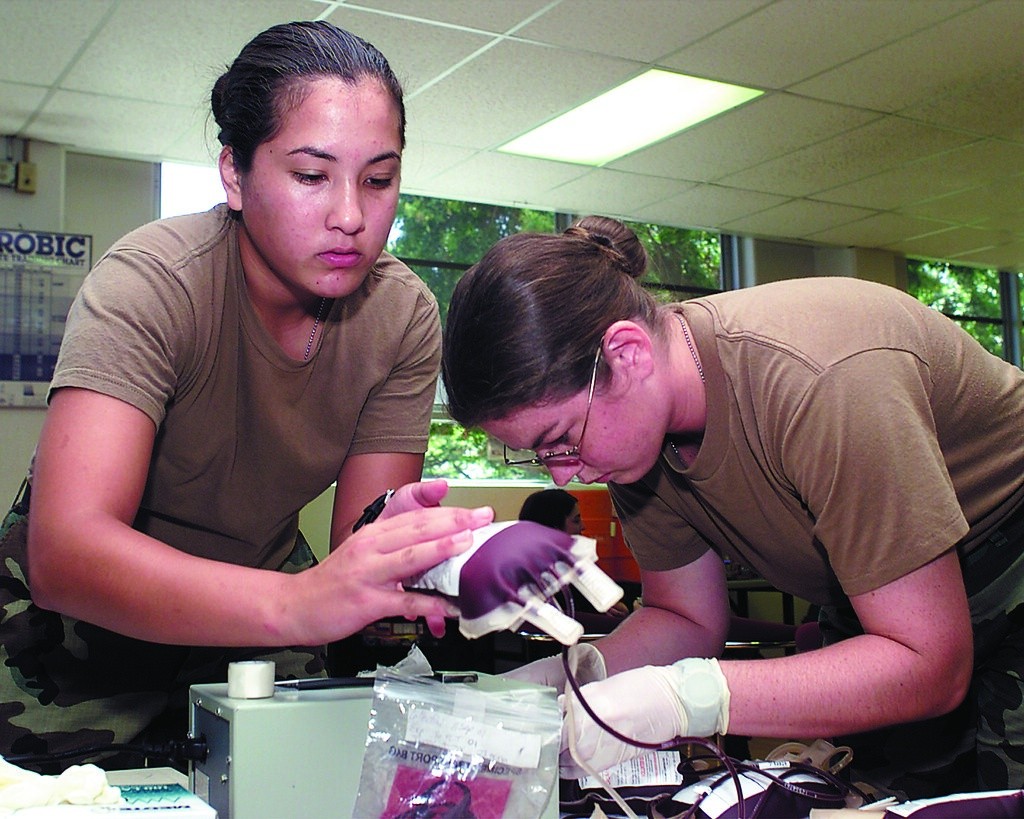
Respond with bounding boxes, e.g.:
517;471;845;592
516;489;827;653
441;215;1023;800
0;21;494;789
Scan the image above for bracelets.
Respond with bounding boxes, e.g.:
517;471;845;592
351;488;396;533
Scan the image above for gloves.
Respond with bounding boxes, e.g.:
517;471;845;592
490;643;729;774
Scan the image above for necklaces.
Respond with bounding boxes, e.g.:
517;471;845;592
666;316;708;466
303;293;328;363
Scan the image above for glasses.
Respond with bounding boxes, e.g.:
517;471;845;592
504;338;605;470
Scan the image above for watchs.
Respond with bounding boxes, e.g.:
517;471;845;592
674;657;721;739
